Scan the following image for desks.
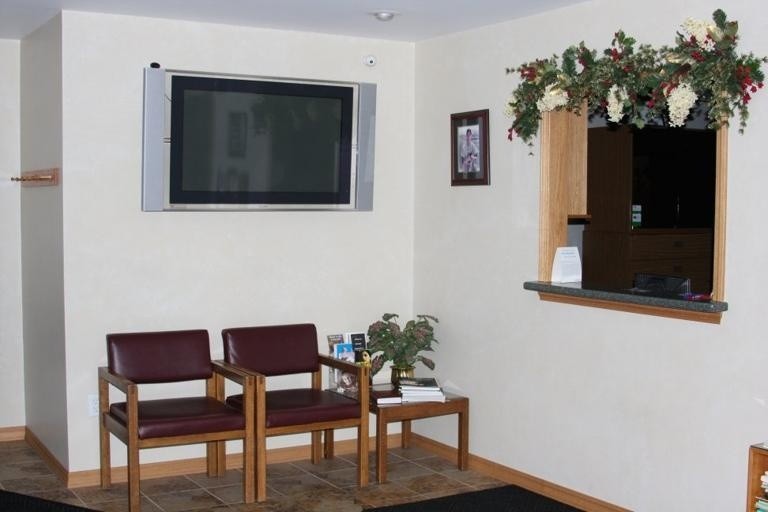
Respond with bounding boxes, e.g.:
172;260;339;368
324;379;469;484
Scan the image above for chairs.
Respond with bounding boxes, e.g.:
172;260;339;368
216;324;370;504
99;329;257;512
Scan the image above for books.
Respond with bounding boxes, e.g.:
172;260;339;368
369;375;445;405
327;333;372;394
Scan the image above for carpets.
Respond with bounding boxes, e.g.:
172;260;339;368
362;485;586;512
0;489;104;512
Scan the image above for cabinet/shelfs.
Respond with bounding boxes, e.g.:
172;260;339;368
746;441;768;512
583;126;714;295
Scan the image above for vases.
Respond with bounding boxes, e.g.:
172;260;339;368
390;365;416;391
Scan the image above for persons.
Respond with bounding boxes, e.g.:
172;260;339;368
458;126;479;172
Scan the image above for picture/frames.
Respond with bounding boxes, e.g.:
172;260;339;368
450;109;491;186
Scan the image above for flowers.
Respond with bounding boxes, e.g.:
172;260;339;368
505;9;768;157
367;313;440;376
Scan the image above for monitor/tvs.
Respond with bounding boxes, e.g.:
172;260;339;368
164;70;359;210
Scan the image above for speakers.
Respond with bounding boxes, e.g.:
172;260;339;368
142;67;164;212
356;82;377;210
